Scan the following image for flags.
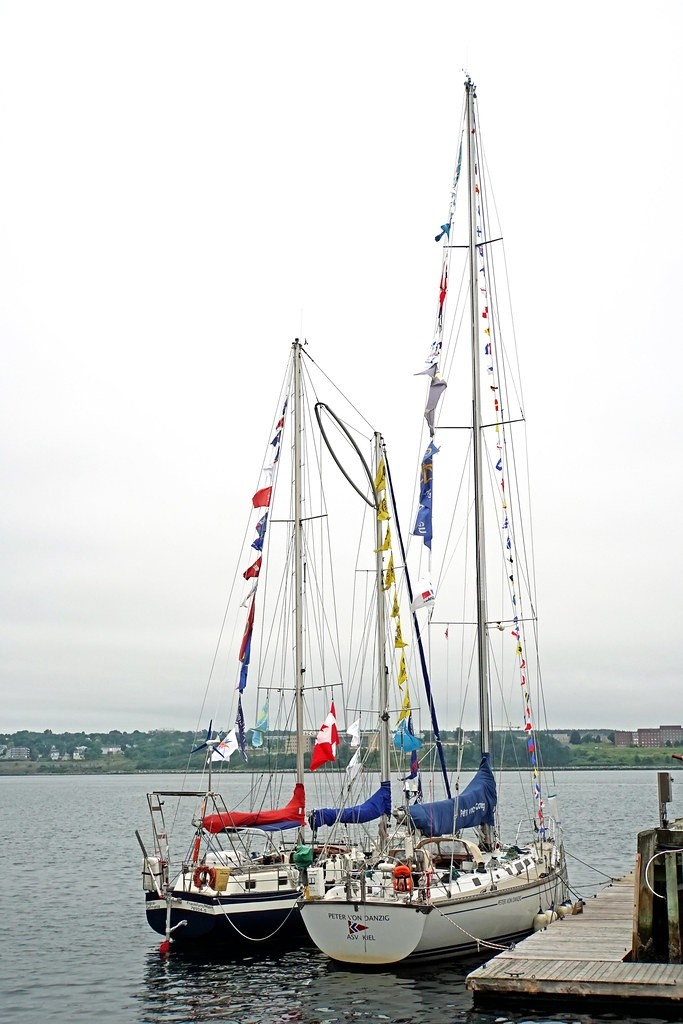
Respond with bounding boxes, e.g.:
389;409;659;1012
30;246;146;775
234;127;546;833
308;702;340;772
207;727;239;764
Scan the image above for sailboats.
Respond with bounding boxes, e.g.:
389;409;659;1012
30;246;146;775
291;73;573;968
120;337;406;954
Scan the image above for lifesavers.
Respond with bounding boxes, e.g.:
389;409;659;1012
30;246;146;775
194;864;216;890
393;866;412;890
